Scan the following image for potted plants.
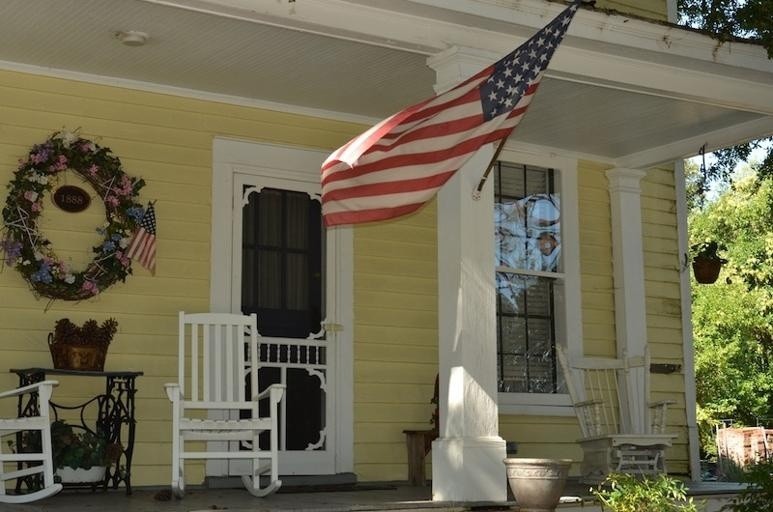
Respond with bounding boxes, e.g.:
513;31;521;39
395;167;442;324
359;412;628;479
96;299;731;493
689;237;728;285
46;316;119;371
37;421;122;485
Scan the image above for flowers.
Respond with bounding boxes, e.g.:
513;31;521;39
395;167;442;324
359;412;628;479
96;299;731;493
2;125;151;313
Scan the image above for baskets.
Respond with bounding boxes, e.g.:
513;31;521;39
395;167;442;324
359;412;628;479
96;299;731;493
48;333;105;371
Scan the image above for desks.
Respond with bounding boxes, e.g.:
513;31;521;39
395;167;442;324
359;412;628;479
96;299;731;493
402;429;439;488
14;365;155;487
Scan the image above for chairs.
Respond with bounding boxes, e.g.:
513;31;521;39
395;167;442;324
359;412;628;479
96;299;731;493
554;343;679;492
165;310;282;502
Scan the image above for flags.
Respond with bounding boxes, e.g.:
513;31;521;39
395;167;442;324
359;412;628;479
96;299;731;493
122;198;159;279
319;0;583;230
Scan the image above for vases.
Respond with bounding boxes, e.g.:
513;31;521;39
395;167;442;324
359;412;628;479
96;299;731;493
502;457;572;509
